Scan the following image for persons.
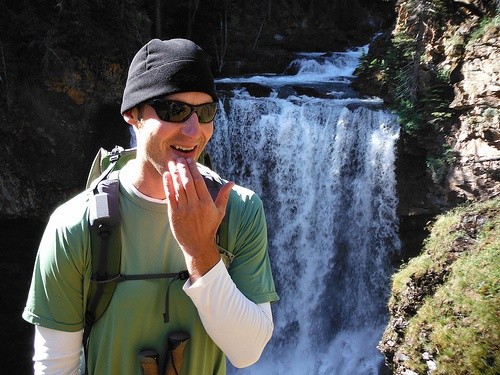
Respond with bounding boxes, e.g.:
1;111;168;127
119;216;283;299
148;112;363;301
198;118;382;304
22;37;281;374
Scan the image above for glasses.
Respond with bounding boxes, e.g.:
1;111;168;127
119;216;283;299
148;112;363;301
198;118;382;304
144;93;219;124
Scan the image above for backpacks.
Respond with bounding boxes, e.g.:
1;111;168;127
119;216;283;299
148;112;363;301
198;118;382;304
81;147;224;346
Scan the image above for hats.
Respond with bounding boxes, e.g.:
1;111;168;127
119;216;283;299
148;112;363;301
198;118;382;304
120;37;220;113
